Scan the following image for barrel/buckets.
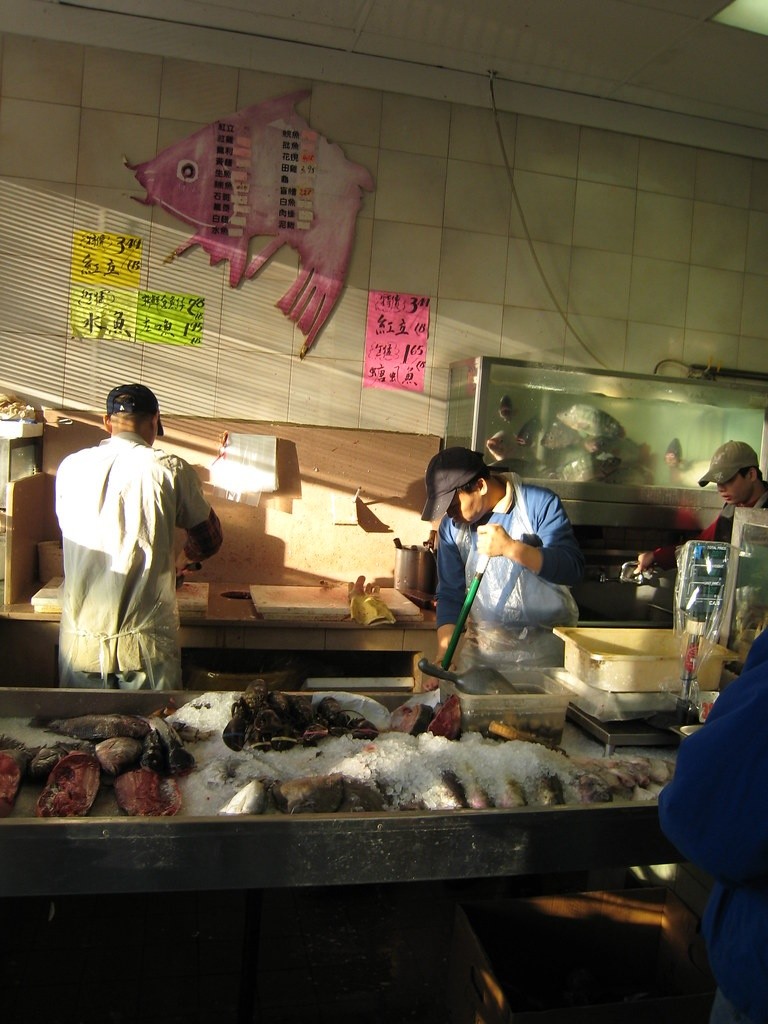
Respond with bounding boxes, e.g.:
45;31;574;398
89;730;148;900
395;546;438;605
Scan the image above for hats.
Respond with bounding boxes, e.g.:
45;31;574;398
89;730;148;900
106;384;163;436
698;441;759;487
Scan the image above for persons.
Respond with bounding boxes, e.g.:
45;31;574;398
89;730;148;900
54;383;223;693
696;440;768;544
421;446;586;693
658;627;767;1024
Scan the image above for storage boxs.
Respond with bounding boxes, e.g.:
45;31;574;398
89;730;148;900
444;884;718;1024
551;626;743;693
718;506;768;692
442;354;768;509
438;670;579;746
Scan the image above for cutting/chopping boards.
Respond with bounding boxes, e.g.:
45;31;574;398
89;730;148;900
31;575;210;613
248;583;424;622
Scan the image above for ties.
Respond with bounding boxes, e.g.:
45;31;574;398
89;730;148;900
421;447;484;521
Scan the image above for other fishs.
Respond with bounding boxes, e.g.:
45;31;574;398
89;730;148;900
27;678;676;812
